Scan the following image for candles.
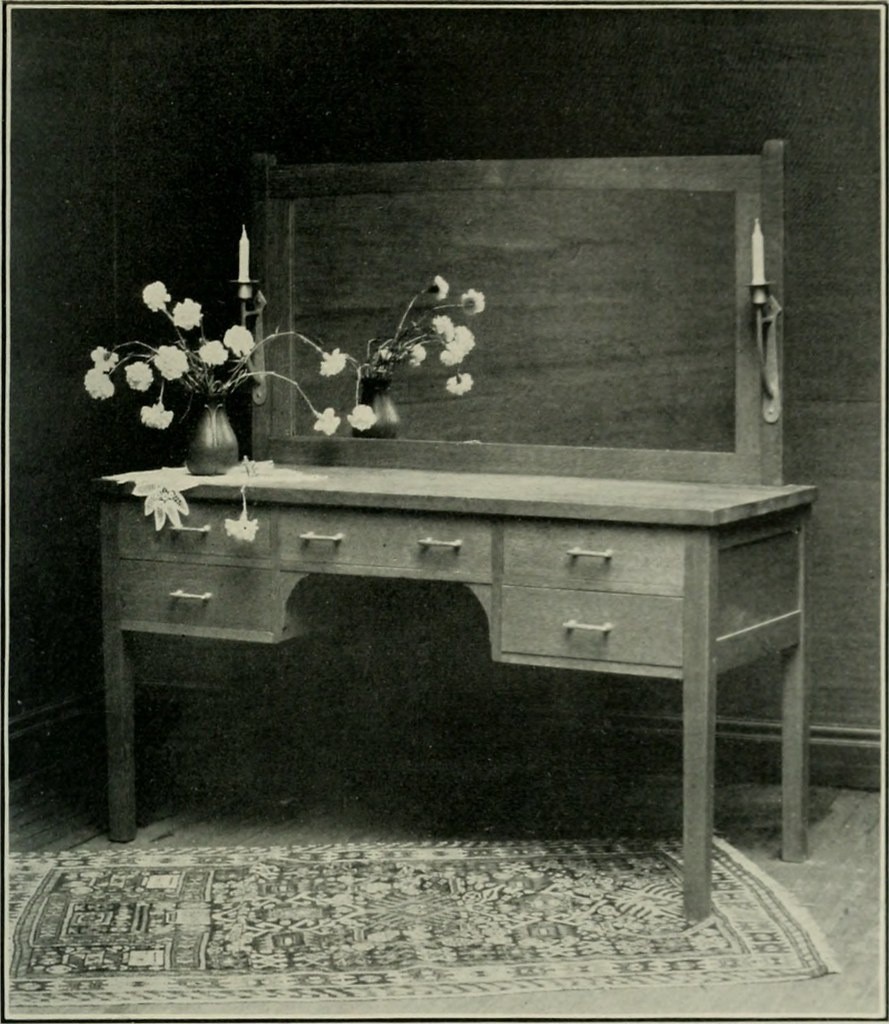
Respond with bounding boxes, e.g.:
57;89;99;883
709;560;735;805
751;217;765;284
238;224;250;283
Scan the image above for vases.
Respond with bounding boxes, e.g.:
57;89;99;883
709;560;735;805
186;394;245;476
350;370;403;438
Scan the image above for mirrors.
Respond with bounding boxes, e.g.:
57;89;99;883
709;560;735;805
253;137;787;485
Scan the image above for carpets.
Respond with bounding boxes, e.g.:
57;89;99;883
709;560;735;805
5;830;837;1005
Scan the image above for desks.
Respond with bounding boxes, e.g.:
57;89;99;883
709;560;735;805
92;466;819;919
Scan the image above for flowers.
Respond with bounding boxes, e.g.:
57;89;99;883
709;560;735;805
318;275;489;432
84;281;353;546
